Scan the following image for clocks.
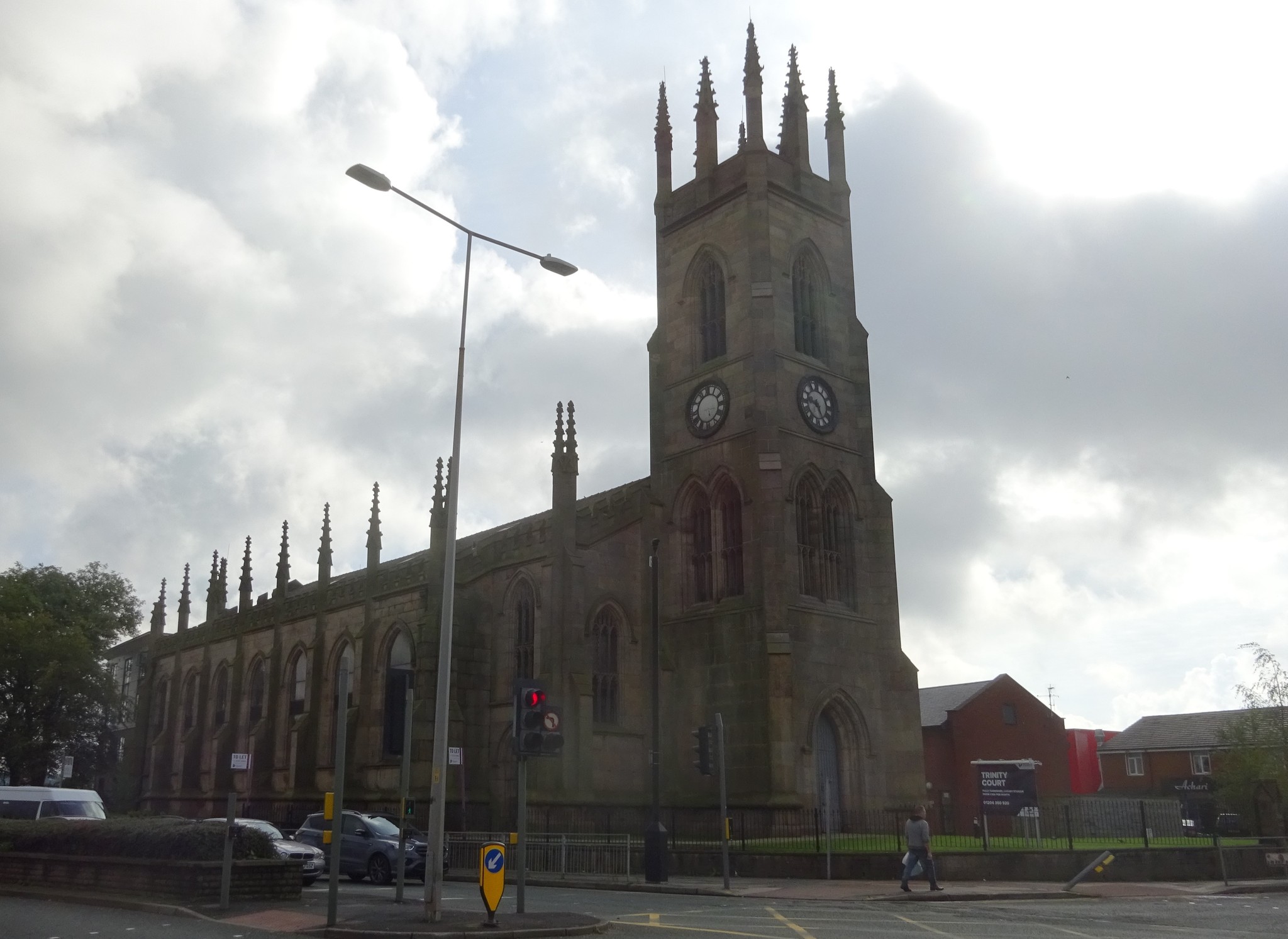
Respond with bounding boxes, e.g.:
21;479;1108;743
687;381;729;437
798;377;838;432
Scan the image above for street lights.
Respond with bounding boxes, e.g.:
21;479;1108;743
348;163;578;921
386;667;414;901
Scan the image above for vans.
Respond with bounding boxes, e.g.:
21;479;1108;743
0;786;107;820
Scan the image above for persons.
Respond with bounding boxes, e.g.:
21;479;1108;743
900;805;945;892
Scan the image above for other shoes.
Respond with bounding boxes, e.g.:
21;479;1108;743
930;883;944;891
900;883;912;892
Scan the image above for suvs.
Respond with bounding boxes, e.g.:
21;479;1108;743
294;810;452;885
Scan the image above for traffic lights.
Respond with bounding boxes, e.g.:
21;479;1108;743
513;678;545;755
541;705;564;755
726;818;734;839
402;797;415;819
692;725;714;774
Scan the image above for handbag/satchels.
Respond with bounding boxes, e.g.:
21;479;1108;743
902;850;922;875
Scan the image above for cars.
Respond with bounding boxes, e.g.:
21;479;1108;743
203;818;326;887
